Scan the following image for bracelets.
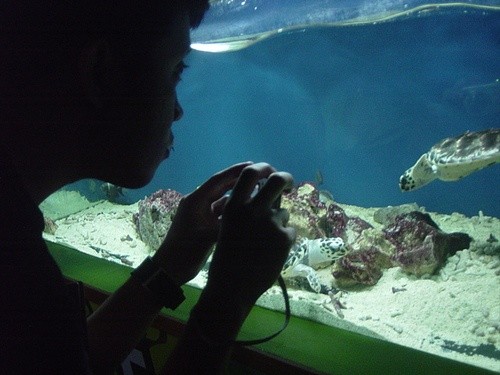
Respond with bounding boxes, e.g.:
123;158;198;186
127;256;187;312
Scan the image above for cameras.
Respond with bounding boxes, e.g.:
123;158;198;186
251;178;281;210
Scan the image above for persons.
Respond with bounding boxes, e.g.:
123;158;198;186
0;2;295;374
